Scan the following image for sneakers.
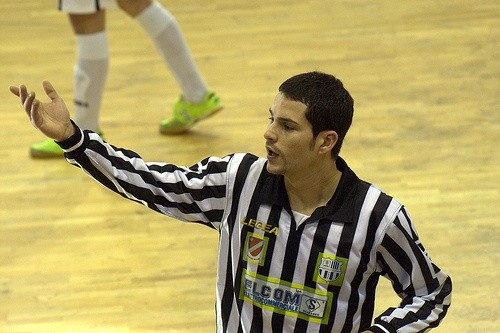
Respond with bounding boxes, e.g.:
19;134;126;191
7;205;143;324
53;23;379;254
160;90;225;133
30;130;107;157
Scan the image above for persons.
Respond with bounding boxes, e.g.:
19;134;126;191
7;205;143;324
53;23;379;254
9;71;452;333
30;0;224;158
